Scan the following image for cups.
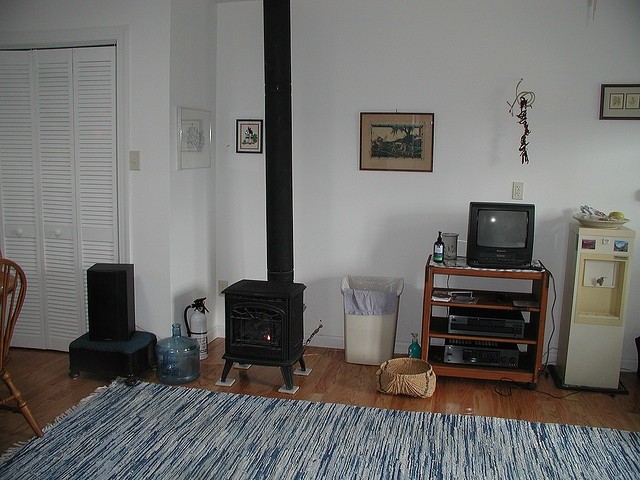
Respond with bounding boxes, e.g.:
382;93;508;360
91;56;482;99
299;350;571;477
443;233;459;260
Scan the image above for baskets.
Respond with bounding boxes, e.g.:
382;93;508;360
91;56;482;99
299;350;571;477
376;358;436;399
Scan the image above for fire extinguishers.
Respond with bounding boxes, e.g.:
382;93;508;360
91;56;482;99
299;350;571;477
185;298;210;362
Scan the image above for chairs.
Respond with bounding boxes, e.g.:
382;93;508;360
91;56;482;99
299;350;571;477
1;256;46;439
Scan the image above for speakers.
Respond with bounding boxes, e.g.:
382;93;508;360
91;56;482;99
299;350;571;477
85;262;136;342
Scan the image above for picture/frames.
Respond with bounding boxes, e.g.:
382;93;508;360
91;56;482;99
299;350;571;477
599;83;640;120
359;111;434;173
236;118;263;154
177;105;213;170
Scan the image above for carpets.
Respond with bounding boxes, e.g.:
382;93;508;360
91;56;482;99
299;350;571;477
3;374;640;480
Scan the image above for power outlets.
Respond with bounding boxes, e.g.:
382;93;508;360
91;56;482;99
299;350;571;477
217;280;229;297
512;182;524;200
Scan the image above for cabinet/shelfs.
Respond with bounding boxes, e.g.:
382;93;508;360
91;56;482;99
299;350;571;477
1;22;130;355
421;253;549;388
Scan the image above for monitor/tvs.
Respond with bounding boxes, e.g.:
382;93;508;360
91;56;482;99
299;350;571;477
466;201;537;269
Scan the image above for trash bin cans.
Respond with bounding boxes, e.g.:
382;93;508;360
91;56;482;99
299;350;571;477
342;274;404;369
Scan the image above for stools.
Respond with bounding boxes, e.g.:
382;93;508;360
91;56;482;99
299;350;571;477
68;332;158;388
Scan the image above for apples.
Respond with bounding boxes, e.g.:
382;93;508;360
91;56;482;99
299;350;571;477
609;212;624;221
